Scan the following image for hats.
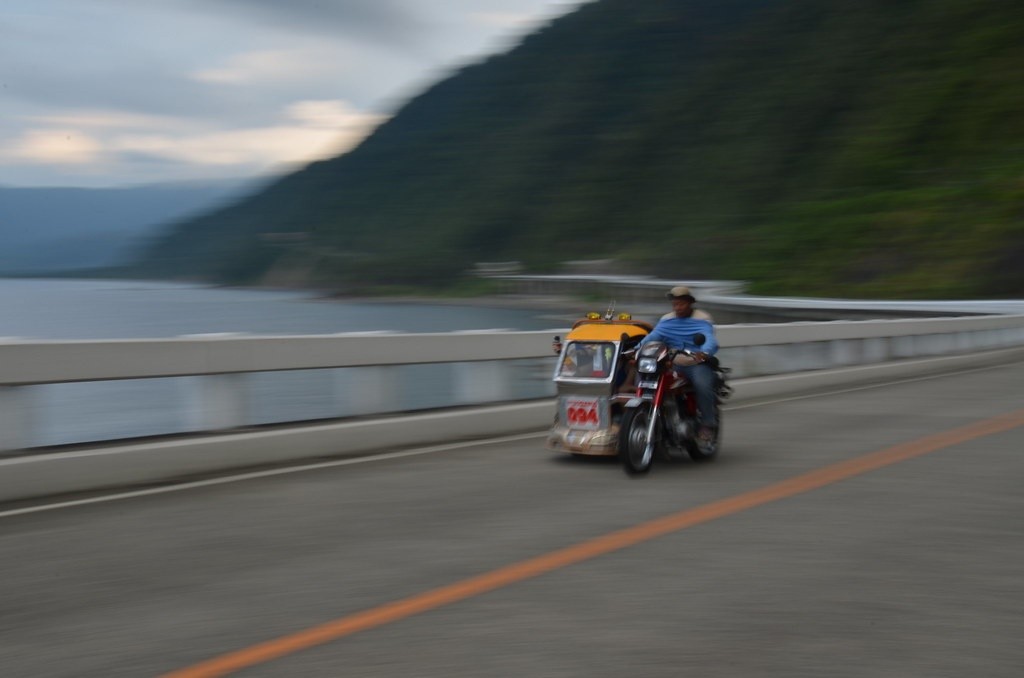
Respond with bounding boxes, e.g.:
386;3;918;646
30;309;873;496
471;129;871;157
665;286;695;304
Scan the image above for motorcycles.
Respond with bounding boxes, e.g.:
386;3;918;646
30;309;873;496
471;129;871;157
544;299;735;478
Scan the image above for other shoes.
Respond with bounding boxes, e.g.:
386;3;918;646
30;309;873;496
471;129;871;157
697;430;713;447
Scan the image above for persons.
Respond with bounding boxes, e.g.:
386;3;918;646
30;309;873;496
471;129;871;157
625;286;720;440
